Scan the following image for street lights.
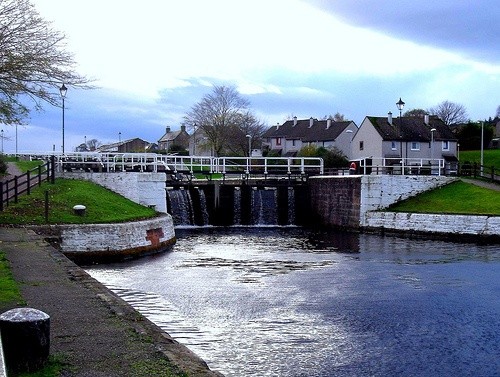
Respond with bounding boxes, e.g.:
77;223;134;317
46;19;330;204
59;82;68;172
430;127;436;173
193;122;198;156
1;129;5;155
246;133;253;173
396;96;406;173
85;135;87;149
346;129;353;160
119;132;122;153
477;119;485;176
14;118;19;161
